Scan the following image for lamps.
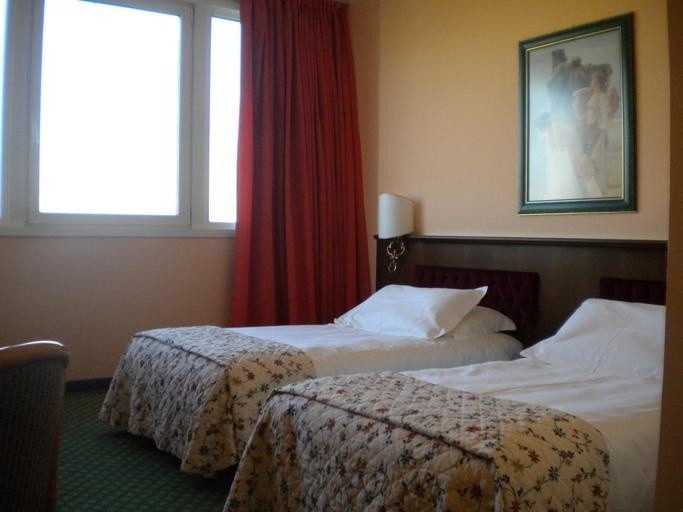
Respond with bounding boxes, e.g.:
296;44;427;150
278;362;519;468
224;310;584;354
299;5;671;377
377;193;414;271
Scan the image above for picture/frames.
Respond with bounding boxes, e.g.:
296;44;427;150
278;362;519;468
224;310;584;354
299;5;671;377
517;11;636;215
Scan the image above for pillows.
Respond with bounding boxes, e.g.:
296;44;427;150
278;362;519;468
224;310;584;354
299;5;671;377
334;285;488;339
439;307;517;336
521;298;666;381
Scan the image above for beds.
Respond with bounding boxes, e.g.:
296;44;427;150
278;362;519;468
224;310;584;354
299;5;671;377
217;276;665;512
100;264;540;476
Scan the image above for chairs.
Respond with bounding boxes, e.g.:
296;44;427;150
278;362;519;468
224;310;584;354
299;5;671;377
0;340;71;512
